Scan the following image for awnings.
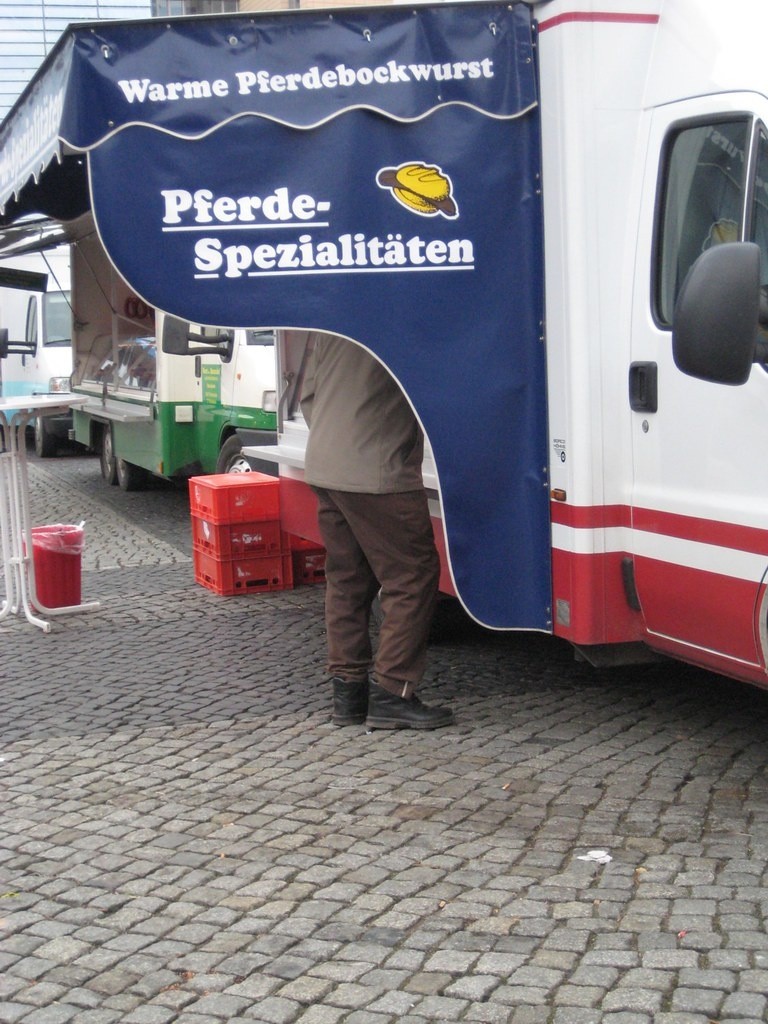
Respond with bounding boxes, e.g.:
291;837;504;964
0;0;552;637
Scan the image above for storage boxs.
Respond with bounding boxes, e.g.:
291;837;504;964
188;472;295;596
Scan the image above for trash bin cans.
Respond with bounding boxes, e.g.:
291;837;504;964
20;523;83;613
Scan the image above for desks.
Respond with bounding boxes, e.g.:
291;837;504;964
0;394;102;634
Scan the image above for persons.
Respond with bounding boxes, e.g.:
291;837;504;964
301;334;454;729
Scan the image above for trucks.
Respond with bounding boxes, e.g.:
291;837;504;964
1;2;768;690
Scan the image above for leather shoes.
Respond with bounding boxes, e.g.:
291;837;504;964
367;679;453;729
332;677;369;726
